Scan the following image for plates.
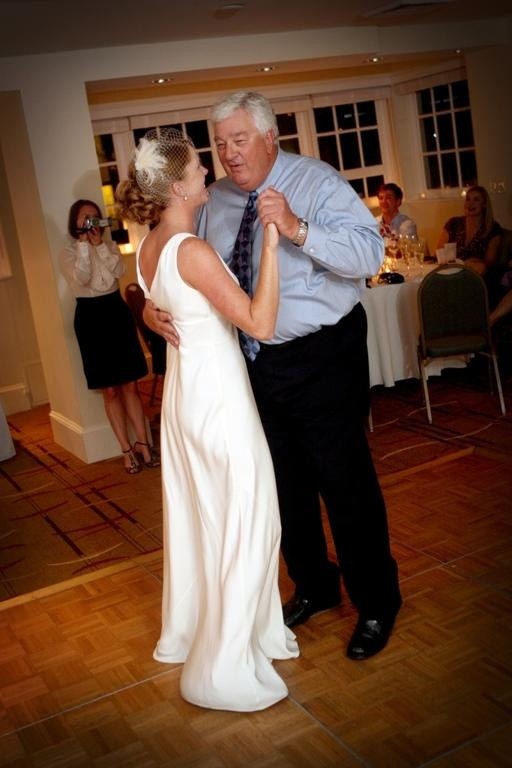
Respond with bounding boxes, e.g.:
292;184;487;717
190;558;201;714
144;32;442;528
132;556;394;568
429;258;466;275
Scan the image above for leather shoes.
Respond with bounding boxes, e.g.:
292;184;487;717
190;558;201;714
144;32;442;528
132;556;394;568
282;579;342;629
346;599;402;660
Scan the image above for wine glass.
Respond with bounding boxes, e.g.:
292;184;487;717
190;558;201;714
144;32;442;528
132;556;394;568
387;234;425;273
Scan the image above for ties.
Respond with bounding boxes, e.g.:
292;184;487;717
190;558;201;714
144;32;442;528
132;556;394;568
231;189;261;364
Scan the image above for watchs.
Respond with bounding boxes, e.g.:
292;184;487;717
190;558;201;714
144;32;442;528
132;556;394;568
294;217;307;245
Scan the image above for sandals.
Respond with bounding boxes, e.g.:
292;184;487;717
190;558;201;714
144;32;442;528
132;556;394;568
133;441;161;468
121;444;142;474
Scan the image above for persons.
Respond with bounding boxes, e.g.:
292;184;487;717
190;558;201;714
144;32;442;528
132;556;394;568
141;90;402;659
114;131;299;713
60;199;161;475
438;186;504;265
375;183;416;258
489;260;512;326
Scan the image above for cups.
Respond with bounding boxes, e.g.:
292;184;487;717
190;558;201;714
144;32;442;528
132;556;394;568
435;242;458;266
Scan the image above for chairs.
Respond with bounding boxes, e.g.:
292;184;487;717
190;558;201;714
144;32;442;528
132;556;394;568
416;264;507;424
125;281;167;407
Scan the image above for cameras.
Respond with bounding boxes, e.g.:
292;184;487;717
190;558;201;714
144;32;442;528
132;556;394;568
84;216;112;229
